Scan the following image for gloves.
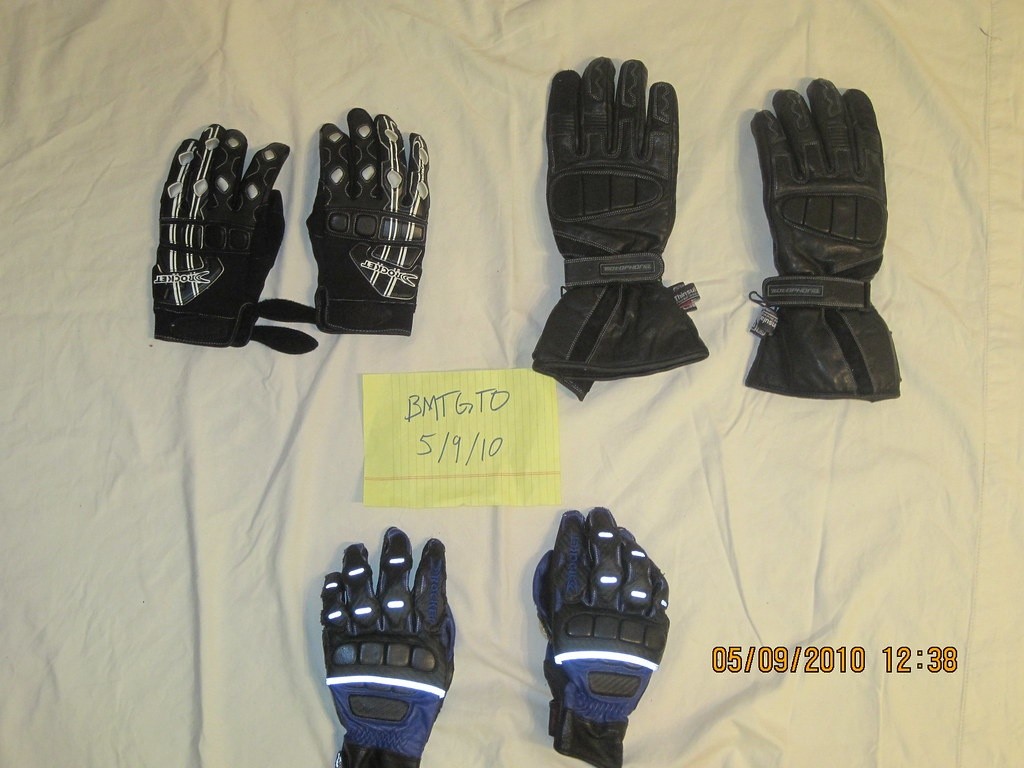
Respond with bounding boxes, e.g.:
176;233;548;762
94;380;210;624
533;507;670;768
320;527;455;768
529;57;710;401
253;108;430;337
153;123;317;354
744;78;903;404
